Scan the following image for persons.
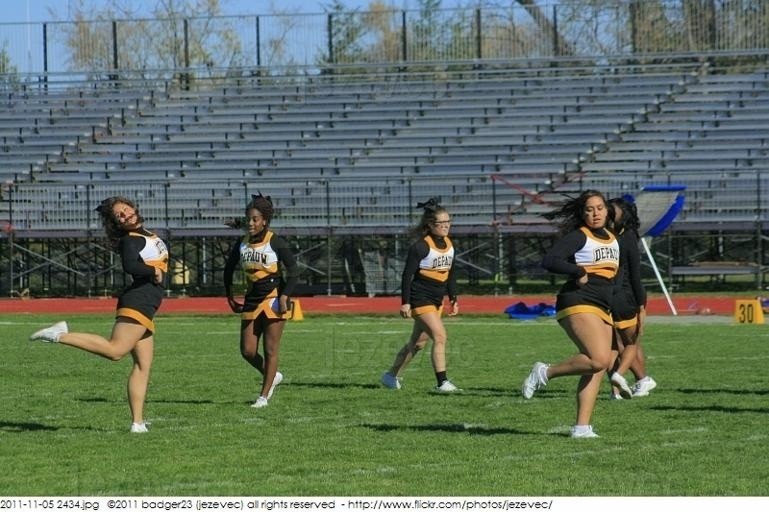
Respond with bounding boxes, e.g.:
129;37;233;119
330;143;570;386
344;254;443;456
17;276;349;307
27;195;170;434
520;188;627;439
603;201;649;399
222;194;300;409
375;196;465;391
607;197;658;398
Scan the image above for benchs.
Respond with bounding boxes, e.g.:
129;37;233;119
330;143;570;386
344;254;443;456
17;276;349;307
668;265;756;289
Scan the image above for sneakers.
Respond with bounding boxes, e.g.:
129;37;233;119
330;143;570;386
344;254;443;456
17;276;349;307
130;422;148;433
438;381;459;391
569;424;599;437
250;397;267;407
381;374;401;389
610;373;657;399
30;320;69;343
266;371;283;398
522;362;546;398
